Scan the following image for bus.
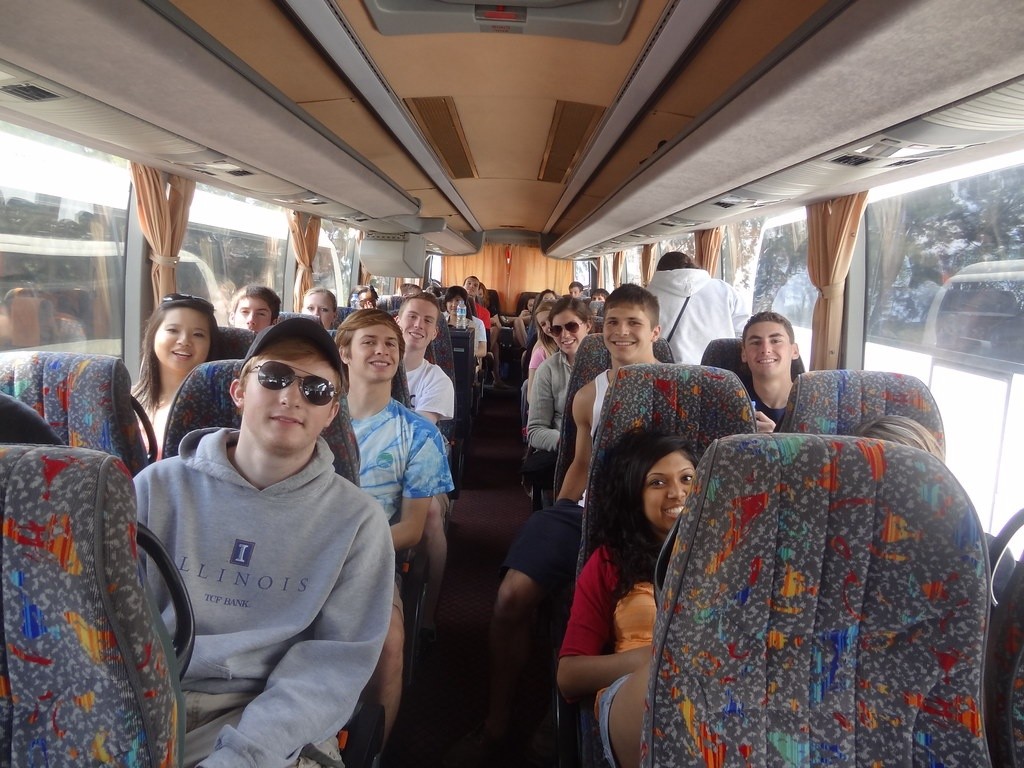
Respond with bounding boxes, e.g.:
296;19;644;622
0;131;346;359
748;150;1024;602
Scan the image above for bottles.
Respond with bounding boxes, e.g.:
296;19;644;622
457;300;466;330
350;294;360;309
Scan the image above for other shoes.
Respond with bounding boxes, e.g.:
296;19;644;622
493;380;508;389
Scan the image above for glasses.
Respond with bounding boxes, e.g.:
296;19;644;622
161;293;214;314
550;322;584;337
246;361;338;406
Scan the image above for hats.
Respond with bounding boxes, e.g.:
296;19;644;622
239;318;343;389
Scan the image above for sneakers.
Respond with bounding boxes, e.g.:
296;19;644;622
525;709;557;765
442;720;513;768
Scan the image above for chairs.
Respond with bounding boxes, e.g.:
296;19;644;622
0;277;501;768
513;289;1024;768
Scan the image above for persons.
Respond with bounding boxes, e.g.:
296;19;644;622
355;276;608;373
336;310;454;764
229;286;281;334
858;417;1015;606
772;269;823;319
527;301;561;402
529;298;592;486
649;251;739;367
556;438;699;768
741;310;798;435
133;317;395;768
399;293;453;423
130;294;218;461
301;288;338;337
442;282;775;768
